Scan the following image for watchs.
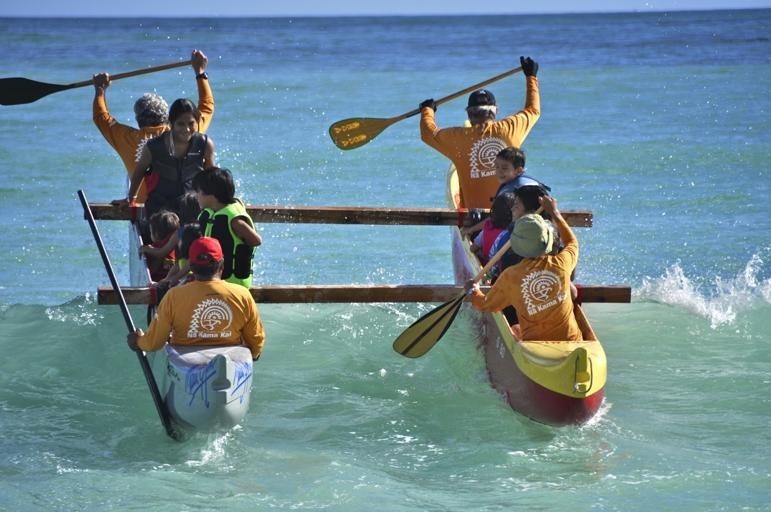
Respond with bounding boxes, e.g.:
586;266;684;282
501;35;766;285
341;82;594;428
196;72;208;80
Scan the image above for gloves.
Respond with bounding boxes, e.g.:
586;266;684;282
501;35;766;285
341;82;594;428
420;99;437;113
520;56;538;77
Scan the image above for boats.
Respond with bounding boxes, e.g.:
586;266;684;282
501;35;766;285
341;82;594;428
451;203;607;428
117;161;255;428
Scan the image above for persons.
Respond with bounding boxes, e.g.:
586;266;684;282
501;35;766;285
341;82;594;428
138;167;263;290
464;195;584;341
462;146;561;284
419;56;541;208
93;49;214;203
127;237;265;362
109;98;216;245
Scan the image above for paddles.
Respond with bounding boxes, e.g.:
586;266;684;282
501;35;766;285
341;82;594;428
1;61;192;105
330;68;523;151
392;206;544;359
77;188;175;434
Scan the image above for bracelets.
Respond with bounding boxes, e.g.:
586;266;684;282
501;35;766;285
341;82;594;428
127;194;138;199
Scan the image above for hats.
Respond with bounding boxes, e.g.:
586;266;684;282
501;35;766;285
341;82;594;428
509;213;553;257
467;89;495;106
188;237;224;265
